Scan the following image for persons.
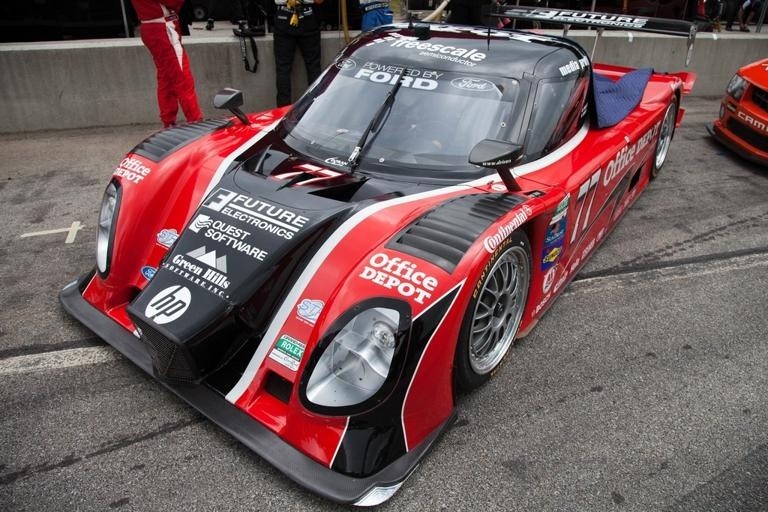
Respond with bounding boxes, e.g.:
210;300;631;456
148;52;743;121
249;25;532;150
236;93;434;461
357;0;394;34
703;0;724;33
724;1;760;33
270;0;326;111
129;0;205;128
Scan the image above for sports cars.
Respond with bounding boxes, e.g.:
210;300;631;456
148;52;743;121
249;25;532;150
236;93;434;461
55;4;699;508
709;61;767;173
